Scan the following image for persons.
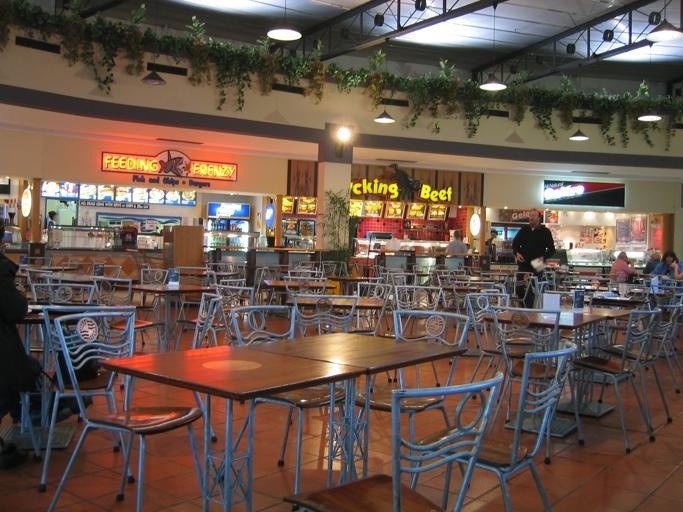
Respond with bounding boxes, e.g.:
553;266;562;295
446;230;468;253
606;252;635;282
512;209;556;308
486;230;499;245
0;223;44;468
643;255;661;274
655;251;678;274
47;211;57;228
671;262;683;280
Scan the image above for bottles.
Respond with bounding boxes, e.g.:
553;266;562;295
210;221;243;250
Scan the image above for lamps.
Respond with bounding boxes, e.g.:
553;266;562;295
266;0;303;42
479;0;507;91
141;1;166;86
637;42;662;122
568;66;589;142
373;39;395;123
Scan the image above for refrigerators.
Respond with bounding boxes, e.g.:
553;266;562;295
204;202;250;253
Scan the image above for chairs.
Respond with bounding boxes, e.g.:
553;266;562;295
10;257;679;510
